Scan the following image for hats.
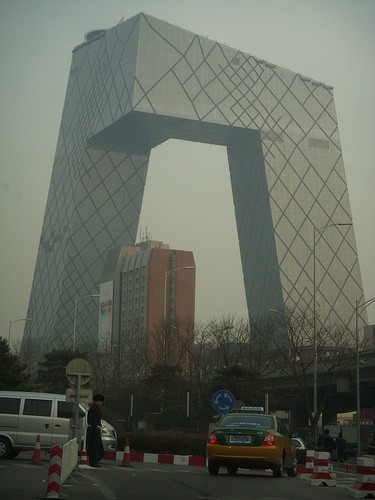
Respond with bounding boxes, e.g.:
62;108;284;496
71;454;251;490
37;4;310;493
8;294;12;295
93;395;104;401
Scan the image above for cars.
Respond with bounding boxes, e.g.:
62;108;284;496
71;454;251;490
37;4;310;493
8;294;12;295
206;406;301;478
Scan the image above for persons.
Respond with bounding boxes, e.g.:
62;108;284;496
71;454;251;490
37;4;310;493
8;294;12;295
335;432;346;461
317;428;335;452
85;394;105;467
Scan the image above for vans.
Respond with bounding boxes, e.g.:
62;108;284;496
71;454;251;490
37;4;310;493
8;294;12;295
0;390;118;461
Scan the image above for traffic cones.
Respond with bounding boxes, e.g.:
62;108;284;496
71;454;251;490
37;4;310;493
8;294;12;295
118;438;134;467
26;434;45;465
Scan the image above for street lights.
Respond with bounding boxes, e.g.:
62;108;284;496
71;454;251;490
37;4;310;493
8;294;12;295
161;266;197;364
198;326;234;408
73;294;101;355
7;318;31;346
312;223;354;452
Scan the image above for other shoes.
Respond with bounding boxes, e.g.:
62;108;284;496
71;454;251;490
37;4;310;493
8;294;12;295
92;464;101;467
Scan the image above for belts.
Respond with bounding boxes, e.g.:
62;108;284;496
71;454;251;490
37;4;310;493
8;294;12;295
88;425;101;429
